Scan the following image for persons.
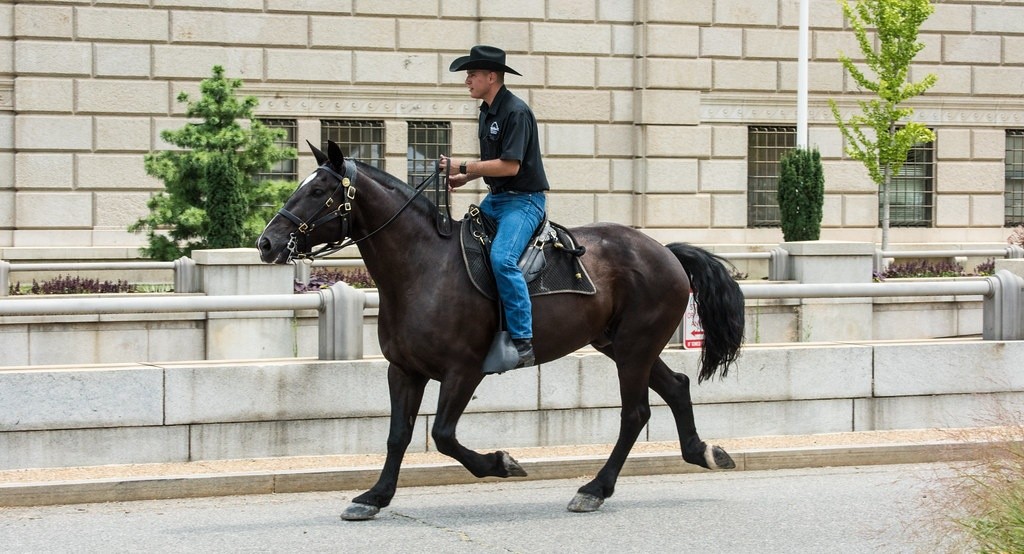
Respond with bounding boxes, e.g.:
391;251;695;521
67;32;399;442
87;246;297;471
438;46;551;371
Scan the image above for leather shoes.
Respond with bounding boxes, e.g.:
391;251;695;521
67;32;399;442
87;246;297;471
512;338;535;368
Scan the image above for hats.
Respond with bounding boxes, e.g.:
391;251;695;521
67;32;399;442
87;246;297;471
449;46;522;77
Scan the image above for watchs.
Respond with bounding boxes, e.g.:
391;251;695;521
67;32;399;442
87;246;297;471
459;161;467;174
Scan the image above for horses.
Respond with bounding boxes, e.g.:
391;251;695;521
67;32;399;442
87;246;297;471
257;139;745;520
